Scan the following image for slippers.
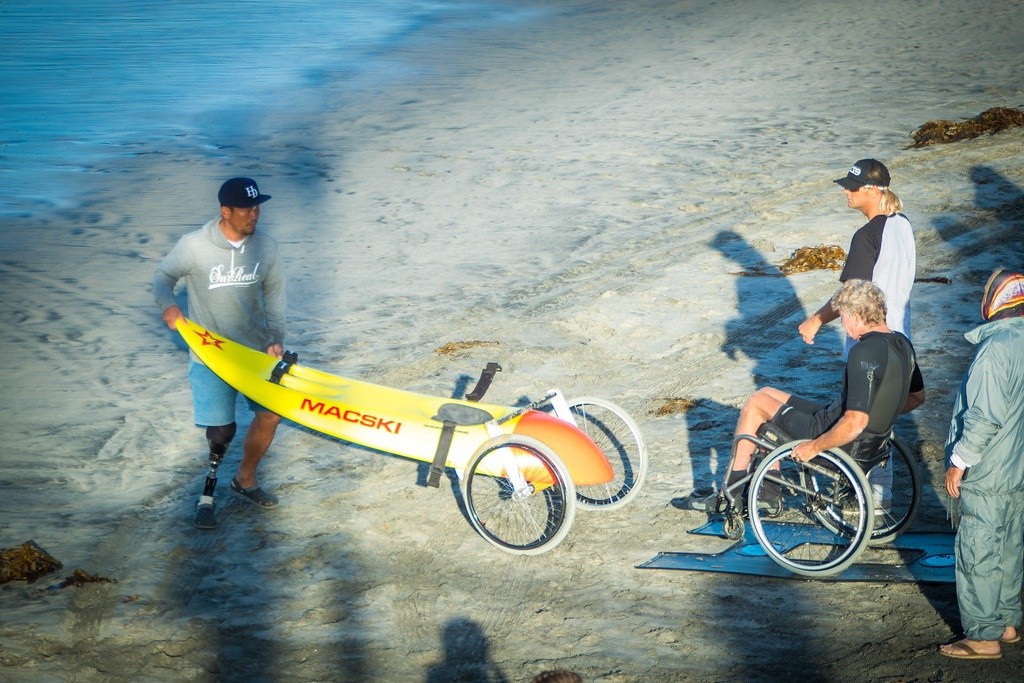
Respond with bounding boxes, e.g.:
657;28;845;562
939;641;1002;660
999;635;1021;643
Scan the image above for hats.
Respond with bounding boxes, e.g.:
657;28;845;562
218;178;272;208
833;158;891;189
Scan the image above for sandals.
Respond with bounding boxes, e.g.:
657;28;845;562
230;475;280;509
193;500;219;529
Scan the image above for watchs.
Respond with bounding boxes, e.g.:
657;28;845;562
949;460;955;467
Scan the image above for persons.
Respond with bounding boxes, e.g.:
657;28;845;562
797;159;916;516
692;278;926;510
943;267;1024;659
152;177;288;529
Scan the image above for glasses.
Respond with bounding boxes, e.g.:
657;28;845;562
845;187;859;192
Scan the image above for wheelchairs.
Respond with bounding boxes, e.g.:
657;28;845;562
713;423;924;581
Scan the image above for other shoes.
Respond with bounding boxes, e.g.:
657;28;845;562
842;495;892;515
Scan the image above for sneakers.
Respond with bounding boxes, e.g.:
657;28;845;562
691;485;745;515
742;473;781;509
